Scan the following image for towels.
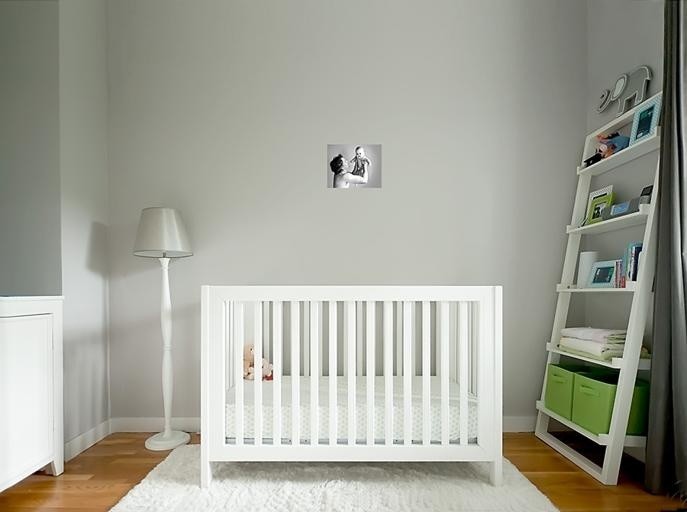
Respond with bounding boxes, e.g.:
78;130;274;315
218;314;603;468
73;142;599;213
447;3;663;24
560;326;650;366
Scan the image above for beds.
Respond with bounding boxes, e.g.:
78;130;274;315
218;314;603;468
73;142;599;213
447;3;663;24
200;283;506;490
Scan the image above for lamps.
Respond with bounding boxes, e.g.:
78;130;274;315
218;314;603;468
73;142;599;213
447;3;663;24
130;207;196;453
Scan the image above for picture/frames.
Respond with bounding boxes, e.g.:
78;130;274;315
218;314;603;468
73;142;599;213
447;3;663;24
628;94;660;144
587;257;623;287
585;184;614;224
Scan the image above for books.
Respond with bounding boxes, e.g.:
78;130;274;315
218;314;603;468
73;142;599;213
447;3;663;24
613;241;642;288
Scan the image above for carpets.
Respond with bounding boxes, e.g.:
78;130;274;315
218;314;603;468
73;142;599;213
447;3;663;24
108;440;559;512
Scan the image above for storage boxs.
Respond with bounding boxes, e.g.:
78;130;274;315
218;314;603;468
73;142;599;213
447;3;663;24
543;363;650;436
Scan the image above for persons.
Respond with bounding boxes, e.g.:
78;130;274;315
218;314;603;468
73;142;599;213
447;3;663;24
347;146;371;178
592;207;600;219
329;153;369;188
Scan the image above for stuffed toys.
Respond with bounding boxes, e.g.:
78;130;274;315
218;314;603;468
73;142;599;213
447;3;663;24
241;340;273;381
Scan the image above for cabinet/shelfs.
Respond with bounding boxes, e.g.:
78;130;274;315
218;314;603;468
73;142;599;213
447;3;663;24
532;90;663;486
